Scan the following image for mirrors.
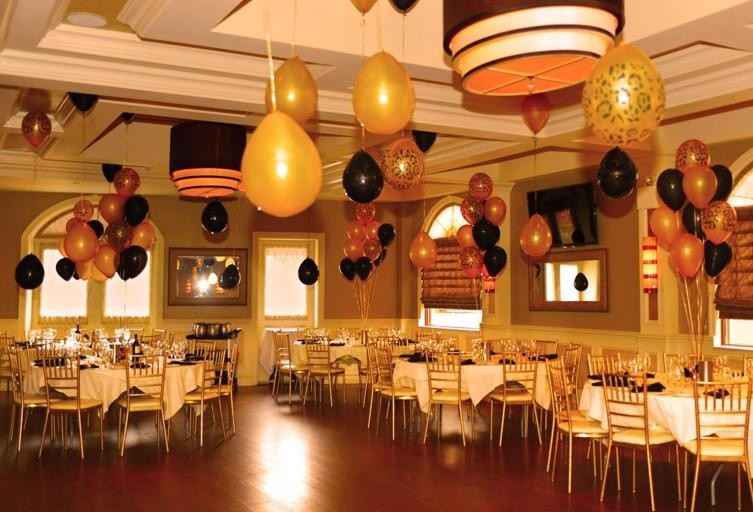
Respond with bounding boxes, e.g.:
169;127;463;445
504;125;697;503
526;247;609;313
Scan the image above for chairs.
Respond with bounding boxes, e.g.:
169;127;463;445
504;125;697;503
0;328;243;460
269;327;752;409
547;359;622;494
682;374;753;511
373;347;419;439
544;356;591;474
366;341;396;427
488;348;543;448
599;369;683;510
423;348;474;447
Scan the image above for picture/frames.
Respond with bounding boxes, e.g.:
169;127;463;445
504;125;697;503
167;246;247;306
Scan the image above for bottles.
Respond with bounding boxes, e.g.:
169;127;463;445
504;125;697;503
75;324;80;342
192;322;231;338
132;334;140;362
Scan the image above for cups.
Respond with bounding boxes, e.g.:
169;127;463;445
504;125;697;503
296;328;304;340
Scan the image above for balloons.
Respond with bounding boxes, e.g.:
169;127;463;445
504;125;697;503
298;257;320;285
15;253;45;290
337;130;440;285
392;0;419;16
520;93;551;133
204;256;234;285
350;0;378;18
69;91;98;115
455;171;507;278
239;112;323;219
581;42;667;147
200;199;229;235
21;111;52;147
264;55;318;124
574;272;588;291
648;138;738;281
519;213;552;257
55;164;155;282
222;265;240;288
351;49;417;136
596;146;639;201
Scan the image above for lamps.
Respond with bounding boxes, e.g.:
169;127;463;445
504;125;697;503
169;122;242;200
444;1;625;98
